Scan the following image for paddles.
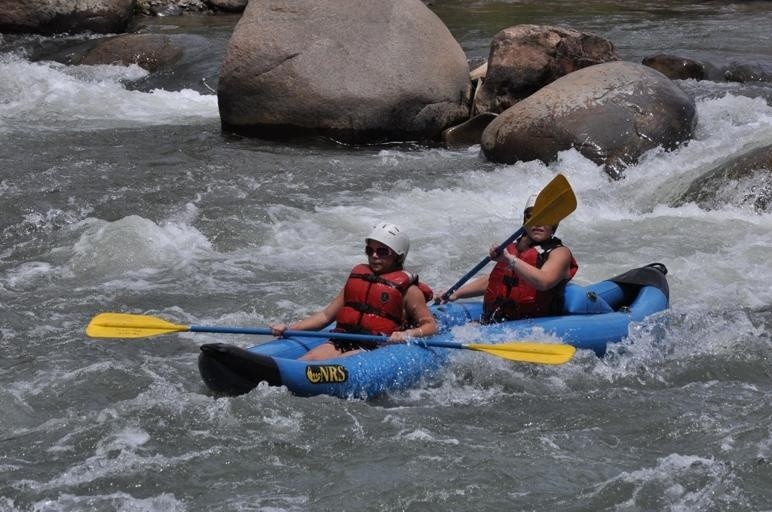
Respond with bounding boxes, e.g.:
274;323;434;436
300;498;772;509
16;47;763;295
440;174;577;300
86;313;576;365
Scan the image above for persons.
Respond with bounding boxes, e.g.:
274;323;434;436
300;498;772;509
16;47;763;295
270;222;437;361
435;189;579;327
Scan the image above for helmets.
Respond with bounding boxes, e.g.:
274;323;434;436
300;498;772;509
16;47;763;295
365;221;410;258
524;195;538;212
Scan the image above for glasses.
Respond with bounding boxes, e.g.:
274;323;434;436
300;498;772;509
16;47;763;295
364;246;389;258
524;213;532;220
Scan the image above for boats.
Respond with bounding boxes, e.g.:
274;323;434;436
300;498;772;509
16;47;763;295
198;263;668;402
